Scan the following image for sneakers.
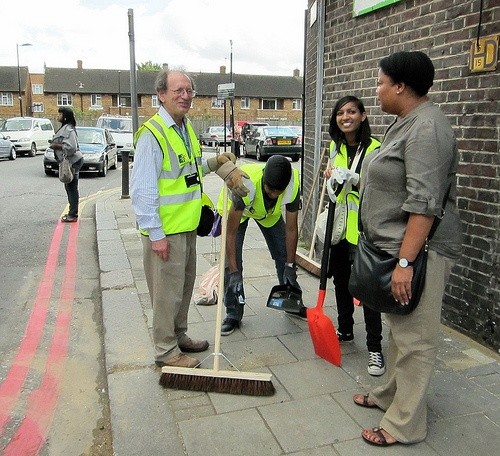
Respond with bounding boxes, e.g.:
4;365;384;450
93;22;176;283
336;330;354;343
367;352;386;376
179;334;209;353
154;351;200;369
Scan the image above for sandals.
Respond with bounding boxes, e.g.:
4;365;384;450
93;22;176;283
361;426;398;446
353;392;379;408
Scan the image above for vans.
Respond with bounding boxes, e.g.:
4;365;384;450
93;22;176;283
0;116;55;157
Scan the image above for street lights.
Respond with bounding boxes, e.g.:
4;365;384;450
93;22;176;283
118;70;122;115
229;38;236;140
16;42;33;117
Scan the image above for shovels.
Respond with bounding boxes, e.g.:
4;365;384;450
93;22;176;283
307;180;346;366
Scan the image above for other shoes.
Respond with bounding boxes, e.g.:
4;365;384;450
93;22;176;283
285;306;310;321
61;214;78;222
221;317;241;336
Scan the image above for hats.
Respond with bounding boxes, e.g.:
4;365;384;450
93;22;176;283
262;155;292;191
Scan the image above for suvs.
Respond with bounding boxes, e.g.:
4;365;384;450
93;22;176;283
241;122;270;145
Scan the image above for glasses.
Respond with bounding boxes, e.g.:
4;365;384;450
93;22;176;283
166;88;197;98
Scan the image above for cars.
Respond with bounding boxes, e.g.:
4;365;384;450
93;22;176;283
287;126;302;145
244;126;302;162
43;126;118;177
0;131;16;160
203;126;232;146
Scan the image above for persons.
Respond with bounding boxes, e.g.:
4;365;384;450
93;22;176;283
323;96;385;375
357;52;462;446
49;106;83;222
132;70;236;369
216;155;300;337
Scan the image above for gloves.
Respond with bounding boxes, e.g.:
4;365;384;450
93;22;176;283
283;262;301;290
327;166;359;203
207;153;236;172
215;161;250;197
223;270;245;309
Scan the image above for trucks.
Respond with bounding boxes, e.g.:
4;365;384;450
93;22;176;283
94;114;134;162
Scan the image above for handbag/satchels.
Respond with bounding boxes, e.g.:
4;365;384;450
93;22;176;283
348;234;428;316
59;154;74;184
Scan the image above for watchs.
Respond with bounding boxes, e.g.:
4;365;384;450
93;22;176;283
285;263;297;269
396;257;414;268
